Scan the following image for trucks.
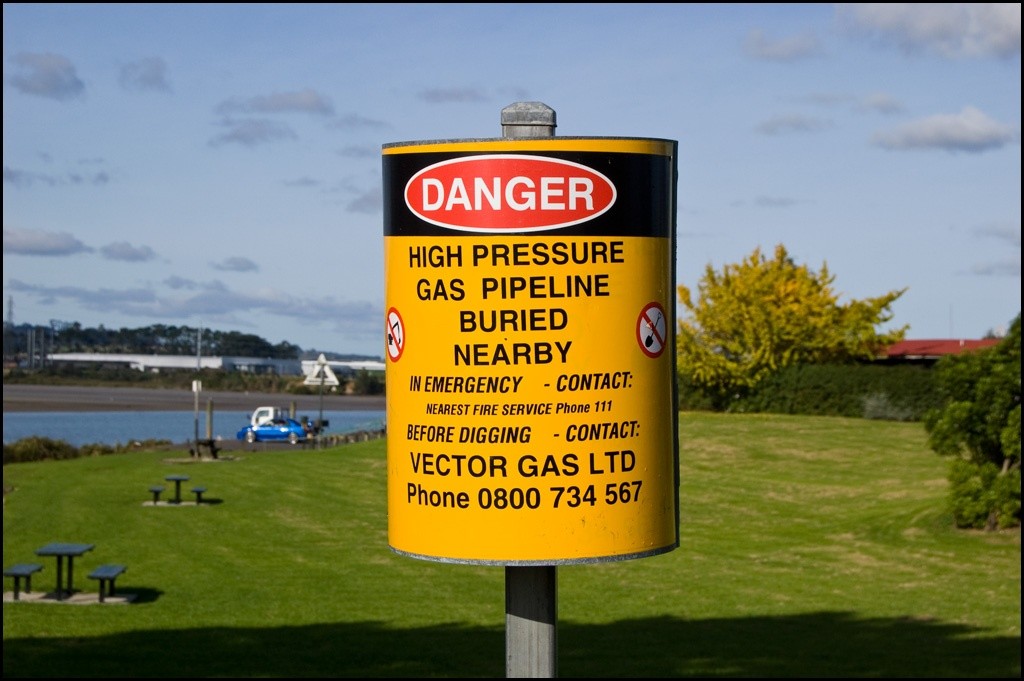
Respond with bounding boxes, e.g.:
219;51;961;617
246;405;329;432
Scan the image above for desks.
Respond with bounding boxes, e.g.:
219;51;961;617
164;476;193;504
34;544;96;601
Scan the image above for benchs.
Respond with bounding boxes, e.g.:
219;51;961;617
149;487;166;502
191;486;208;503
86;565;129;604
5;564;44;600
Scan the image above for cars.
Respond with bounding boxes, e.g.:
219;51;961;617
236;416;311;443
